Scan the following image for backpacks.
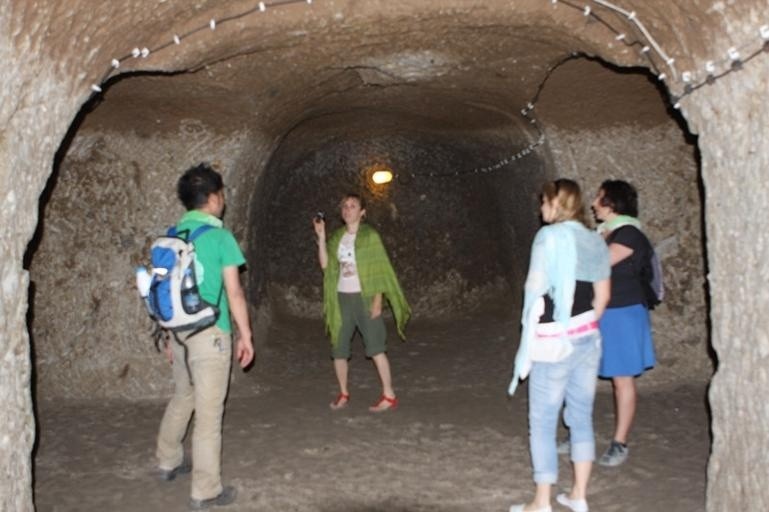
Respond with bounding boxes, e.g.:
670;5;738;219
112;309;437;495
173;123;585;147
136;225;224;331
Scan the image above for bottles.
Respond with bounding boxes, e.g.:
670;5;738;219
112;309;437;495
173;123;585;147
182;268;201;314
134;265;155;315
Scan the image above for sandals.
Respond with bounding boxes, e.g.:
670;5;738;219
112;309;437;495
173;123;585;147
335;393;350;405
370;396;398;412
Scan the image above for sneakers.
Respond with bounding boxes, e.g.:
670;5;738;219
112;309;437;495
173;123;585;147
509;440;628;512
189;486;236;510
154;457;192;482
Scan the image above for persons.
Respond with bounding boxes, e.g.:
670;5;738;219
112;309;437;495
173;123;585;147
313;194;413;412
556;180;664;466
508;178;612;512
151;164;254;512
